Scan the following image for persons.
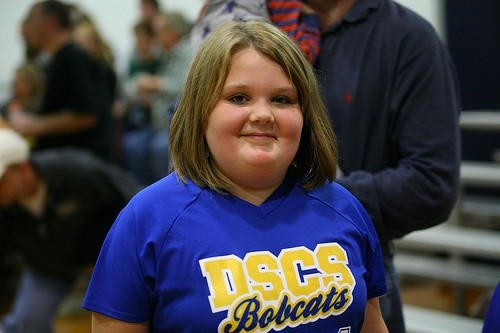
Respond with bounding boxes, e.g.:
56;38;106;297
81;21;389;332
0;0;197;317
482;280;500;333
189;0;462;333
